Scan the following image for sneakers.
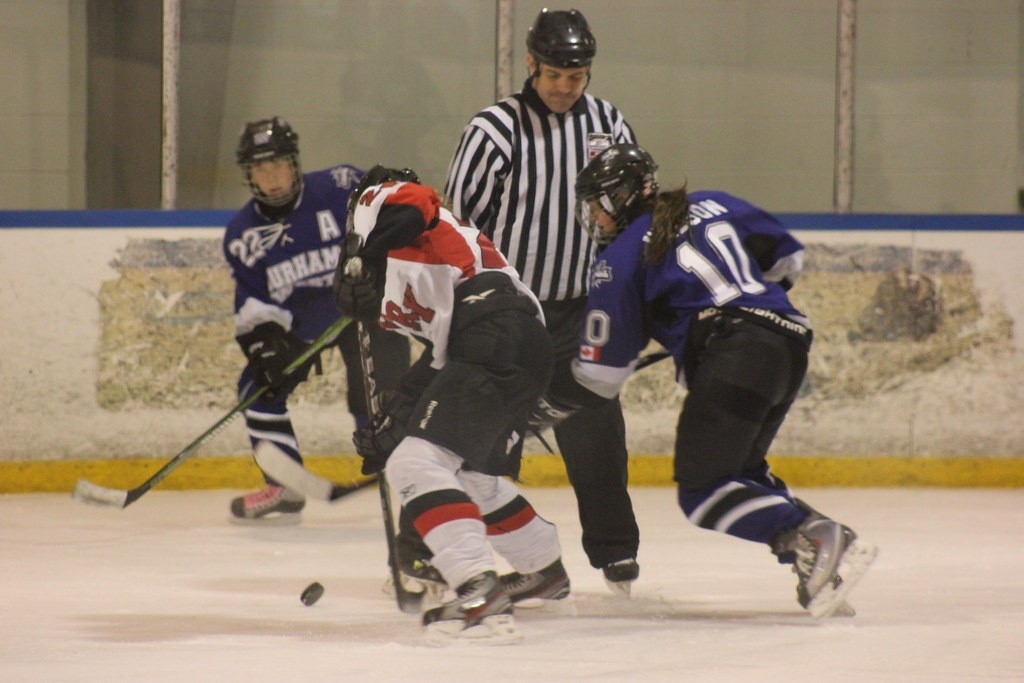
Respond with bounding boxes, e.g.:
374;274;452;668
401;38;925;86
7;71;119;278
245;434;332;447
607;556;641;598
783;509;876;614
419;585;514;647
231;487;303;523
497;565;572;606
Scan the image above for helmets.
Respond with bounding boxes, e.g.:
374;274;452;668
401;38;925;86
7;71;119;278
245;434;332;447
582;144;657;247
239;117;300;205
527;7;596;67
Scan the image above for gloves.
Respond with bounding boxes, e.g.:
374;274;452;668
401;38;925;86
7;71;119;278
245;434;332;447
249;343;278;403
333;256;376;313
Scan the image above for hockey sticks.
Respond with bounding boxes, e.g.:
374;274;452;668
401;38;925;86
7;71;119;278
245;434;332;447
352;313;429;616
73;309;355;513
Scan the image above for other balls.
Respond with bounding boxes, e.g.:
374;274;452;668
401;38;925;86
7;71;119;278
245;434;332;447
300;582;324;607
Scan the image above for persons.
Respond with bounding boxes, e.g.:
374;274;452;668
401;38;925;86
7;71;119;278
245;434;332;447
221;114;386;526
522;141;879;621
439;6;645;597
333;163;570;643
